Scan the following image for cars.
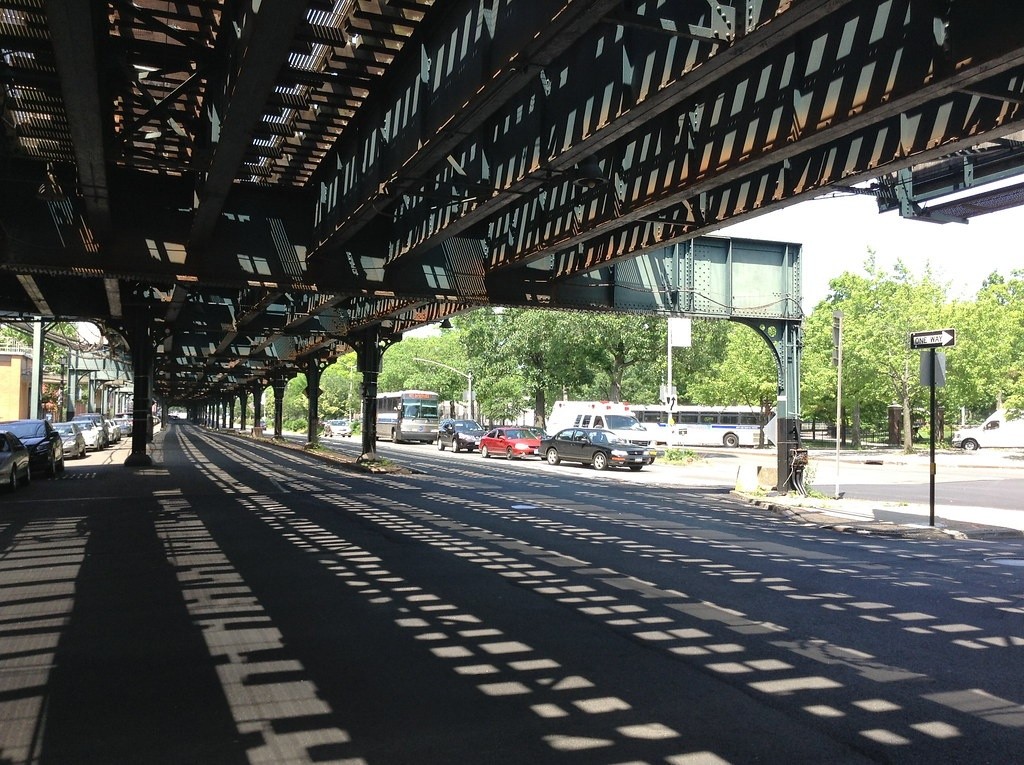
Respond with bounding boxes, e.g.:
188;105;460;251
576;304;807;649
538;428;655;471
51;412;179;462
479;428;543;461
0;431;31;491
260;421;266;429
0;420;66;477
322;420;353;438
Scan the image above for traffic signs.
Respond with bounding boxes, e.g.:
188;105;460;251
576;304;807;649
910;329;954;347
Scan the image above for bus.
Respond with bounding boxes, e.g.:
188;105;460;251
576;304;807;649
361;390;440;444
628;404;774;449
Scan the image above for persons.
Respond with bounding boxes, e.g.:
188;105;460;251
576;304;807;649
517;433;522;438
580;434;590;443
596;434;605;443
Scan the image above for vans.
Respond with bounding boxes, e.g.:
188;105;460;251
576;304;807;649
953;407;1023;452
550;400;655;452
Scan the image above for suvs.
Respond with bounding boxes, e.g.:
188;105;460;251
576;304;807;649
436;418;487;454
